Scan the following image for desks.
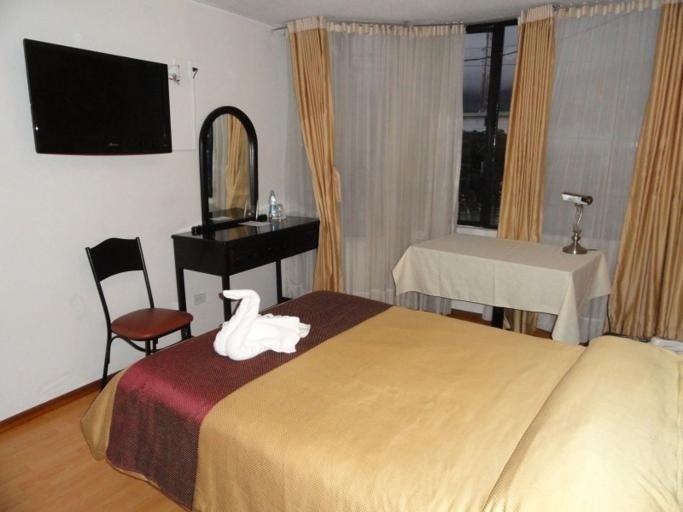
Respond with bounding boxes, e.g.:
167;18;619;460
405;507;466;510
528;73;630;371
172;216;321;336
413;234;601;329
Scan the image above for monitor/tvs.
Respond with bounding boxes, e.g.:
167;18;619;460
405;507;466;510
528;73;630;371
23;38;172;155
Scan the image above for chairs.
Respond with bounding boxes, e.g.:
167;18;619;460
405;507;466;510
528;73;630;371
86;237;193;389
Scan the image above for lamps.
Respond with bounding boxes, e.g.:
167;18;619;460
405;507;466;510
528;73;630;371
561;192;593;254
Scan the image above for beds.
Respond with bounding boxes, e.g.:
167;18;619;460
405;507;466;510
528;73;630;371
119;291;682;511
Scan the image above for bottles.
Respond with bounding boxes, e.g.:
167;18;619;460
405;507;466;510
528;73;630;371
268;191;283;219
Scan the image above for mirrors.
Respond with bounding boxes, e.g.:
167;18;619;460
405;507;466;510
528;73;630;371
200;106;259;229
